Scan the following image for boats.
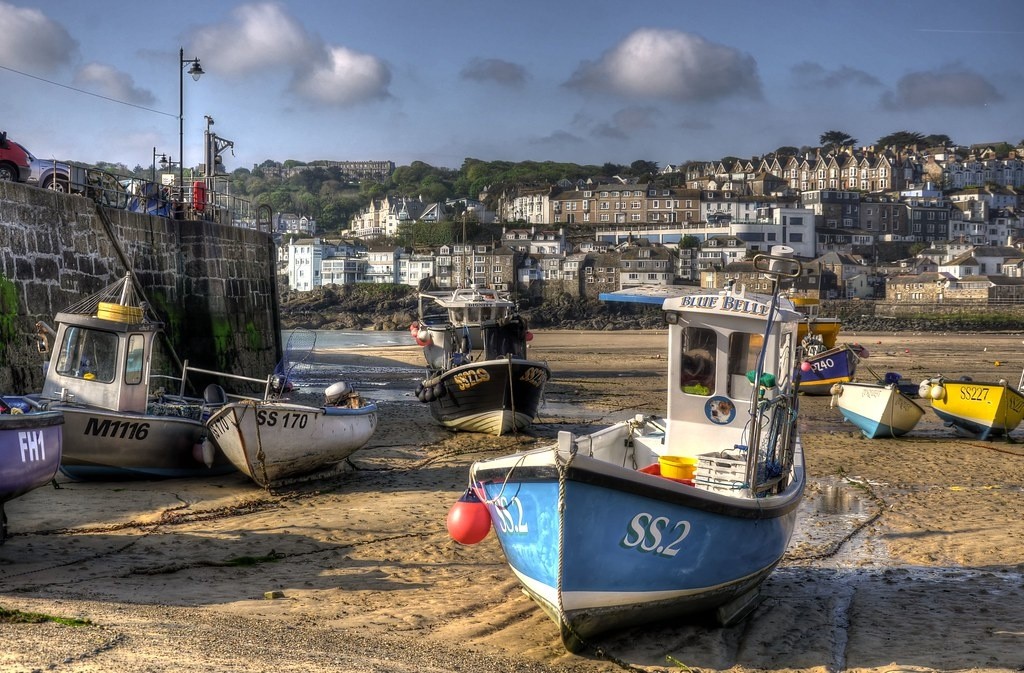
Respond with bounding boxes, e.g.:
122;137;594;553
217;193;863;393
466;245;818;658
793;341;870;397
745;289;843;353
919;372;1024;440
411;281;552;436
830;373;926;440
199;328;384;491
28;268;226;476
0;392;68;506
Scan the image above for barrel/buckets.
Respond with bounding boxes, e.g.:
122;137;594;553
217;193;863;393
884;372;902;384
657;456;697;480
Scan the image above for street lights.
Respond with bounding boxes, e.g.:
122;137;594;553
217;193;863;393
151;146;167;182
176;46;206;214
202;114;214;176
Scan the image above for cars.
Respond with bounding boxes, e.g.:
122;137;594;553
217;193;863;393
11;141;75;193
0;130;33;184
